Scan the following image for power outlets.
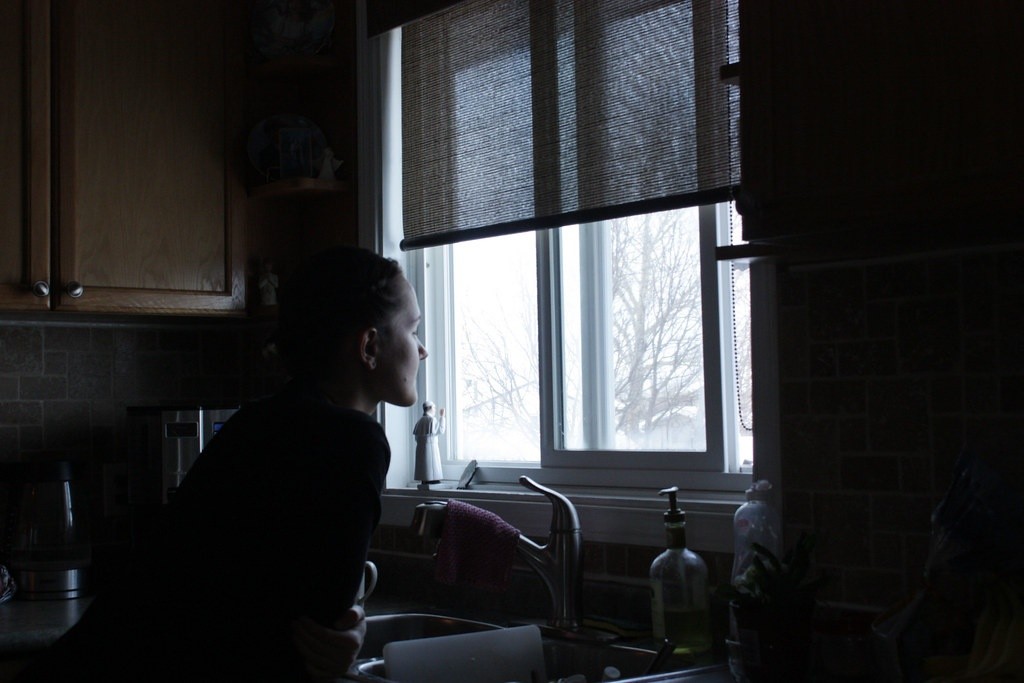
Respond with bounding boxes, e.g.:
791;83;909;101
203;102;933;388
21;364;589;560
104;463;129;518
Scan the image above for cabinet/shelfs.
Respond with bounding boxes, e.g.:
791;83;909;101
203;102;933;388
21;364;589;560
0;1;249;318
248;50;349;322
712;30;1023;273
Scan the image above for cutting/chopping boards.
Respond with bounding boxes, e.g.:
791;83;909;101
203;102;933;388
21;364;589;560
383;625;547;683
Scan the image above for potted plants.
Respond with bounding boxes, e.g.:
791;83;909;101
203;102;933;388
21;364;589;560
714;531;820;683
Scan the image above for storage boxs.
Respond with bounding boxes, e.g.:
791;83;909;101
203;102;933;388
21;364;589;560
815;595;894;679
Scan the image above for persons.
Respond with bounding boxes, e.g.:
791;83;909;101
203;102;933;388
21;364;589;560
12;246;424;683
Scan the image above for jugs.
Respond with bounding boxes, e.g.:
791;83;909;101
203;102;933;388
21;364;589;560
13;461;97;600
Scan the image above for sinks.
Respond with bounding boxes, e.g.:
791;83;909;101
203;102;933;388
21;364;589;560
347;627;729;683
357;606;515;665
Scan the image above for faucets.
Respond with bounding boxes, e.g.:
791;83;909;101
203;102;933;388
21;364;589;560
408;472;586;633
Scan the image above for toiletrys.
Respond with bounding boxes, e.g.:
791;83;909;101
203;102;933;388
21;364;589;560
647;482;716;654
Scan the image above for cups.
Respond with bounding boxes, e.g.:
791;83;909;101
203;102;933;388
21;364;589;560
355;561;378;610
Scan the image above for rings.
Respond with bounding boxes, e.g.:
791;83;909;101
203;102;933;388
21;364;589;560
321;659;329;669
